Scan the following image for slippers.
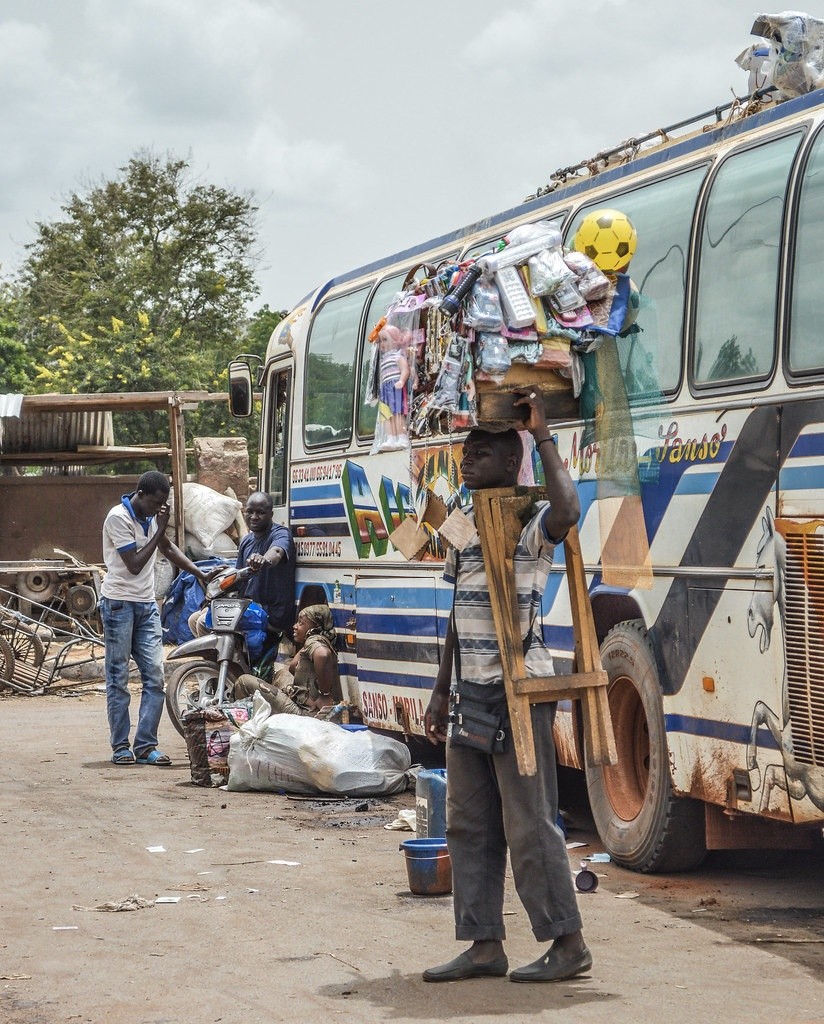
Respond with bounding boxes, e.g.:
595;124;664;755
111;749;135;764
136;749;172;765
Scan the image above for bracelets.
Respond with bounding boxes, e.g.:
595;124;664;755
318;689;331;696
536;437;556;452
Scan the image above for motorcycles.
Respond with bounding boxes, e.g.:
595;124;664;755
166;563;261;743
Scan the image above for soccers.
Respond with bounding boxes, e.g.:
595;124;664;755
572;207;638;276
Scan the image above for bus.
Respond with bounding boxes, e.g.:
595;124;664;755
225;14;823;880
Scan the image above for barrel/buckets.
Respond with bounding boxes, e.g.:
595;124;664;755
413;767;449;839
338;723;369;732
397;837;454;894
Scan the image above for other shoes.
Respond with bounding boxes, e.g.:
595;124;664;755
510;944;592;983
423;951;507;986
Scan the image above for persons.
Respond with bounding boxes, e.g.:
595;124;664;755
187;491;296;694
99;470;230;765
423;385;594;986
233;605;343;722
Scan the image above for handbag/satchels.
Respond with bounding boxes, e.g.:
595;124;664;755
183;701;254;788
452;680;511;755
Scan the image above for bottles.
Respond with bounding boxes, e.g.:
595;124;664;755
748;47;772;94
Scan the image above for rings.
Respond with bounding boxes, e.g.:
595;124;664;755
530;392;537;399
